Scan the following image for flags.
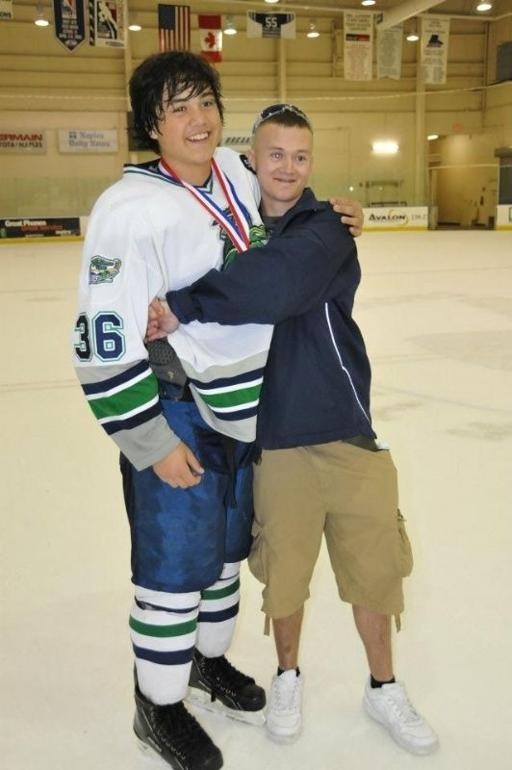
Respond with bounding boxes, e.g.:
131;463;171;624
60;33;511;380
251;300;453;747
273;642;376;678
50;0;88;54
246;10;297;40
198;13;223;64
89;1;126;49
157;3;190;53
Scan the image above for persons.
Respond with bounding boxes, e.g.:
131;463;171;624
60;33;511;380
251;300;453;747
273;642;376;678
146;102;439;757
72;49;365;770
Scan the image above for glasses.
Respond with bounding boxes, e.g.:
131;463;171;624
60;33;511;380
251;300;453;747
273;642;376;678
252;104;312;133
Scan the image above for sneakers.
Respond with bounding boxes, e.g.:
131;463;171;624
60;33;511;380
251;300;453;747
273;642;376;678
266;672;303;745
189;646;266;711
362;677;440;755
134;701;223;770
